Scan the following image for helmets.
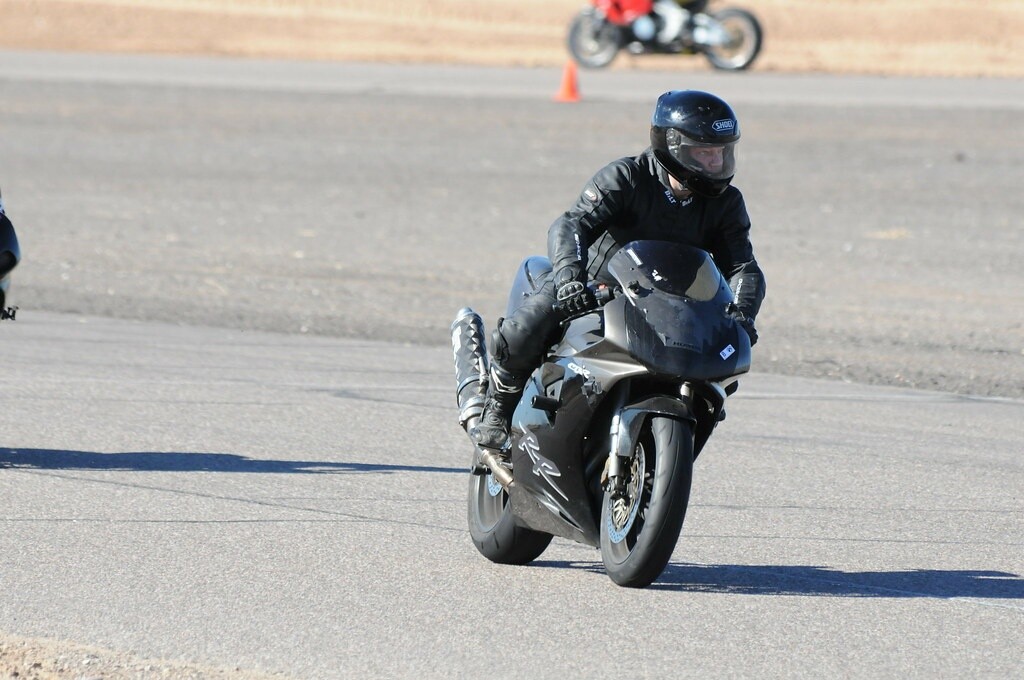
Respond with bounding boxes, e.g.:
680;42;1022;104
652;91;741;203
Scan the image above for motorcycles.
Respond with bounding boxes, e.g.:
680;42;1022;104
567;1;762;70
449;234;756;588
0;183;22;325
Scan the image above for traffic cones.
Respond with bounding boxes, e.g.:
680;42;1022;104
552;60;582;105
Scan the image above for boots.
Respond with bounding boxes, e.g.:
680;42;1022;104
474;360;526;448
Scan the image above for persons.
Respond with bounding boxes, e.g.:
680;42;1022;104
473;91;767;448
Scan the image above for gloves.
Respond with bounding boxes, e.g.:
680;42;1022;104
731;305;759;347
552;267;597;318
